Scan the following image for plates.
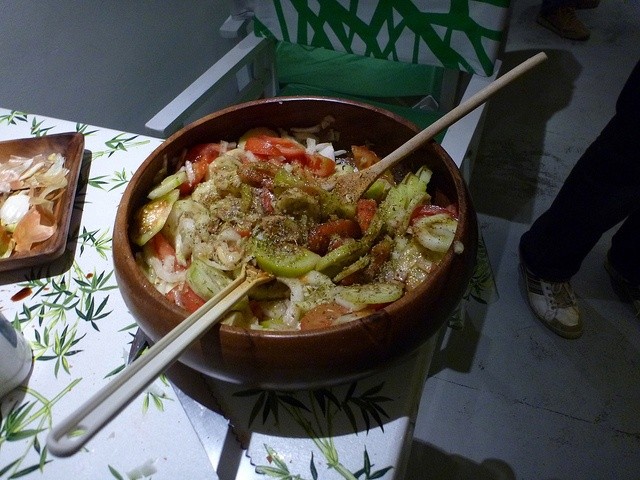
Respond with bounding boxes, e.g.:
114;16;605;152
1;132;85;272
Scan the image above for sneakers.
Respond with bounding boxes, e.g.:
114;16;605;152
520;263;585;340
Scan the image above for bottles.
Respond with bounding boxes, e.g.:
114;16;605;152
0;311;33;395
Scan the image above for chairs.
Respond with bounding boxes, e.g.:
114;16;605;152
145;1;508;178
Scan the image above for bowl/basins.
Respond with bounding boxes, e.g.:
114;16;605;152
112;94;481;392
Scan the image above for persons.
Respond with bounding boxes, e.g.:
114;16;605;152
518;52;640;339
